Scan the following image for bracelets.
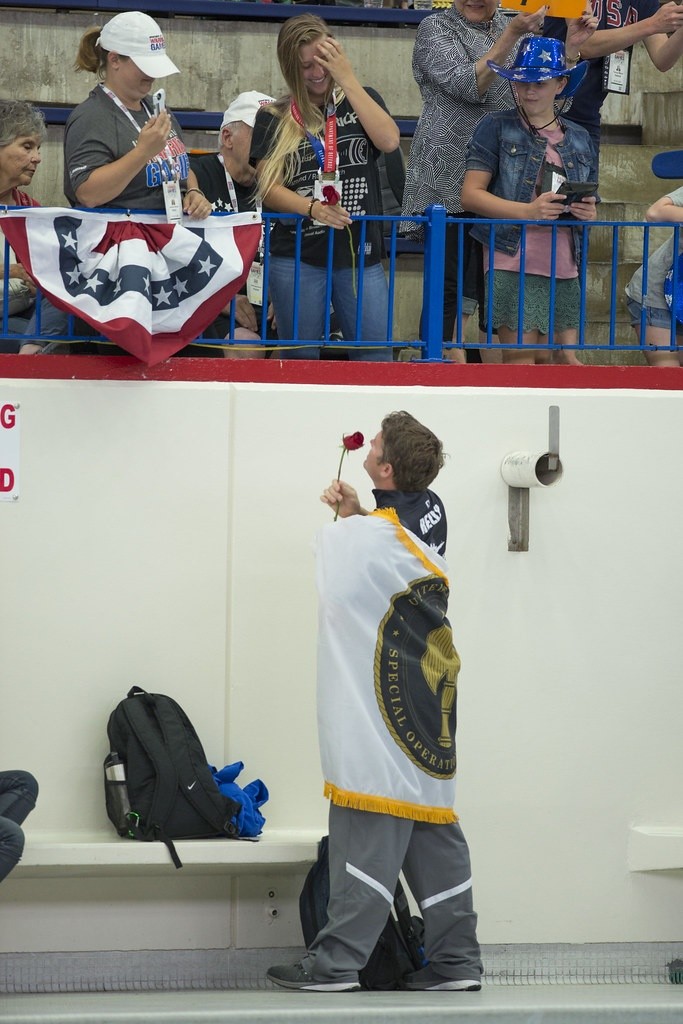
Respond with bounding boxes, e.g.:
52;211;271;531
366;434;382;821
308;198;319;221
185;188;204;197
565;51;580;63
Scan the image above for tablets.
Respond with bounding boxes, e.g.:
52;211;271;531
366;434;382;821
551;180;599;219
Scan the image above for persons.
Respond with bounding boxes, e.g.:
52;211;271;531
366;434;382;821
265;409;484;990
460;37;599;366
624;187;683;368
0;99;90;355
401;0;597;363
188;90;282;360
64;11;212;360
249;14;400;363
539;0;683;156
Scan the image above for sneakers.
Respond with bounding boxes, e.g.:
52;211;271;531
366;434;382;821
265;963;361;991
404;966;481;990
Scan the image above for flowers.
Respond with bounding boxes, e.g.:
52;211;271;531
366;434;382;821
322;184;360;303
326;430;365;523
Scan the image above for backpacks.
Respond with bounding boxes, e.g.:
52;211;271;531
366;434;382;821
299;836;424;990
102;687;259;869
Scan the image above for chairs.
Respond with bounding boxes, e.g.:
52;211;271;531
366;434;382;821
361;85;432;254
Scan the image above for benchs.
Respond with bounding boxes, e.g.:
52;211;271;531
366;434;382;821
14;829;328;870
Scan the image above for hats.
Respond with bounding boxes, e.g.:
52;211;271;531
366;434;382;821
95;11;181;78
220;90;279;129
486;36;589;101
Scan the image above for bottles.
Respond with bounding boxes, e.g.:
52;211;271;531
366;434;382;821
363;0;383;9
413;0;433;10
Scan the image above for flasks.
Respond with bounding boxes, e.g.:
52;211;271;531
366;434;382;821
104;752;132;830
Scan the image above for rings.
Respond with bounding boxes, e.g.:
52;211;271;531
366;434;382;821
538;23;543;27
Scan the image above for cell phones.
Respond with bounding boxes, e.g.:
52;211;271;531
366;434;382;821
153;88;166;119
